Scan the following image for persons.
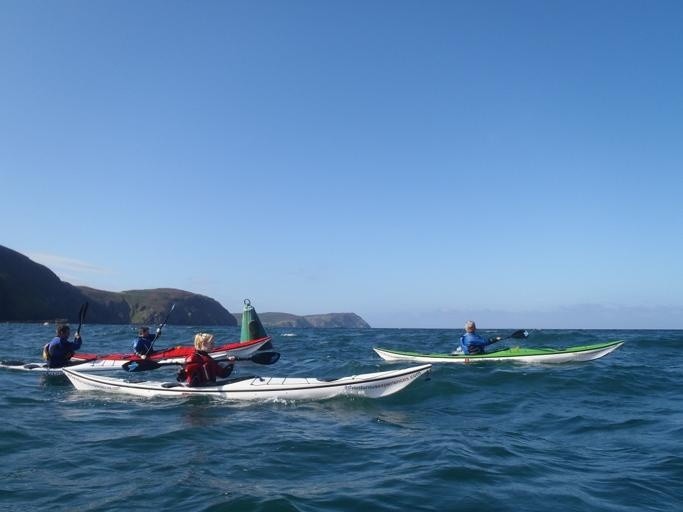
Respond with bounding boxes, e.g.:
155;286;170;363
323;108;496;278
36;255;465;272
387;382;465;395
183;333;236;387
459;321;502;355
47;326;82;368
133;326;161;360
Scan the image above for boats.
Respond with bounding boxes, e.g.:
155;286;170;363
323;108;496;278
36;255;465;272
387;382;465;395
57;364;432;402
366;339;626;369
1;334;280;374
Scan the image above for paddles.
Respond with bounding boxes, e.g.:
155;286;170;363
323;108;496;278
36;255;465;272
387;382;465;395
485;330;529;347
144;304;176;355
73;301;89;342
122;352;280;371
